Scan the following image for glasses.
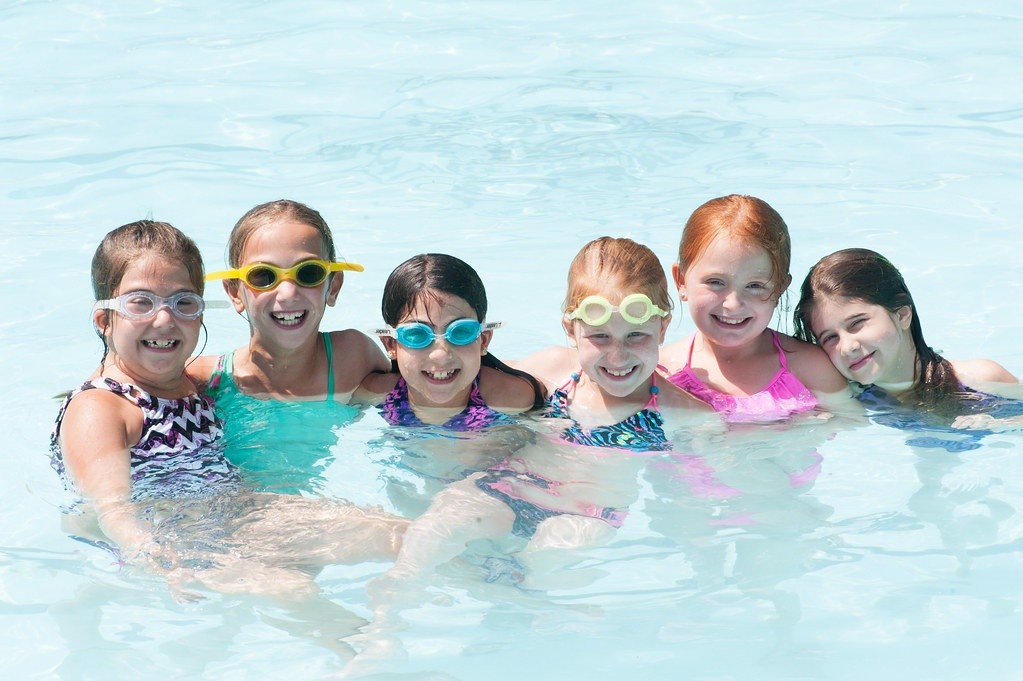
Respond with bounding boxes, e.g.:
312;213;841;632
203;258;365;292
91;292;230;322
374;321;501;349
569;293;667;325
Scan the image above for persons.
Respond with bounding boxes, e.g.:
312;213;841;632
788;248;1020;385
183;198;392;394
345;252;547;416
657;194;846;413
513;235;716;445
50;218;222;483
